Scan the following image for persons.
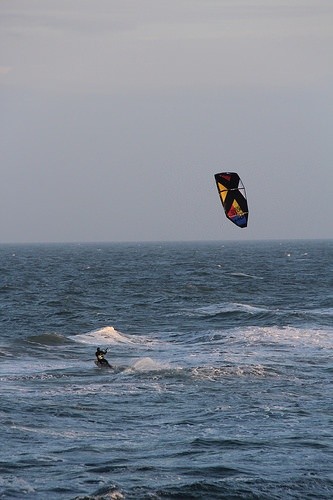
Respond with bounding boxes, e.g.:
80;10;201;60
96;347;109;364
